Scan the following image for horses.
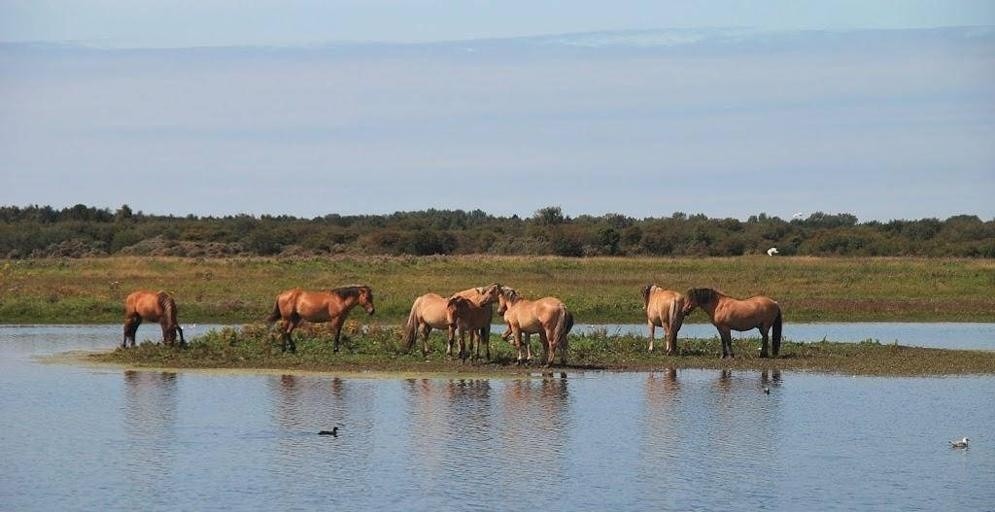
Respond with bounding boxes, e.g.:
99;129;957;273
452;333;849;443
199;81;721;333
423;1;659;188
266;283;375;353
682;287;782;360
640;283;685;356
122;290;185;348
400;282;574;369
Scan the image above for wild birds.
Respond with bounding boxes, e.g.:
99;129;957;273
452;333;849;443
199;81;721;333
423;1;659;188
763;387;769;393
948;437;970;446
317;427;340;435
